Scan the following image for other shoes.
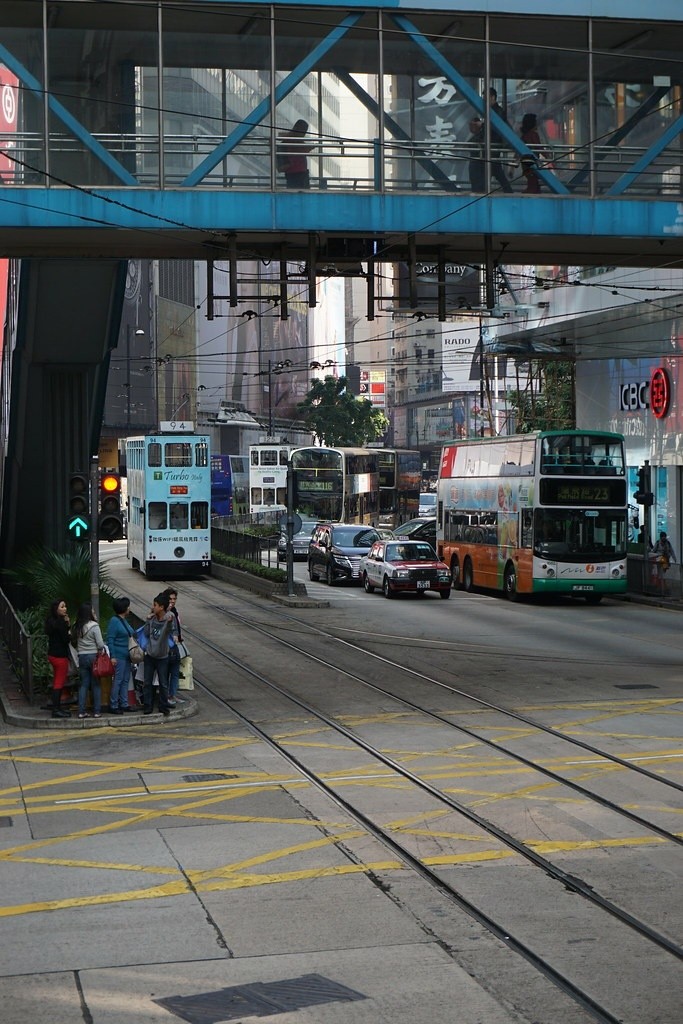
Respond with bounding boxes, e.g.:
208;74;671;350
120;706;139;712
167;698;186;705
78;713;91;719
143;708;153;714
108;708;123;715
94;713;102;718
51;710;70;717
158;707;170;714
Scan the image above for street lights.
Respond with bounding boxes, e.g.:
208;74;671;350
269;362;283;436
127;326;146;436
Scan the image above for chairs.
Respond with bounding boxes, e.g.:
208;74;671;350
541;460;615;475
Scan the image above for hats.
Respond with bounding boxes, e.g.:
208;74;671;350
113;597;131;614
154;593;172;611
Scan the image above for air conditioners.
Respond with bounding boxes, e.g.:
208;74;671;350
413;342;420;346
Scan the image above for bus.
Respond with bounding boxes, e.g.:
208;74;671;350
118;421;211;580
366;448;422;531
248;436;309;527
286;446;379;529
211;455;249;524
437;429;640;601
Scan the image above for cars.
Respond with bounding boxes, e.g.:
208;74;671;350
359;536;452;599
419;492;437;517
278;522;318;561
392;516;436;551
376;529;399;541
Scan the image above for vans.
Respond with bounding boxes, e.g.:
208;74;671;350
308;522;382;586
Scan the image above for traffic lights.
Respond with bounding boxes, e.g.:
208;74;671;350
637;493;654;506
634;469;643;498
100;471;123;543
66;477;91;541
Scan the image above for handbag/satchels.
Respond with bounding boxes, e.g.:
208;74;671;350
128;636;145;664
178;657;194;691
92;646;115;677
174;641;191;659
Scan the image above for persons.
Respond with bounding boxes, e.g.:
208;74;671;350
282;119;315;189
143;587;185;715
43;599;72;718
467;117;482;192
71;602;104;719
507;113;555;194
106;596;138;715
471;86;514;194
638;525;677;574
